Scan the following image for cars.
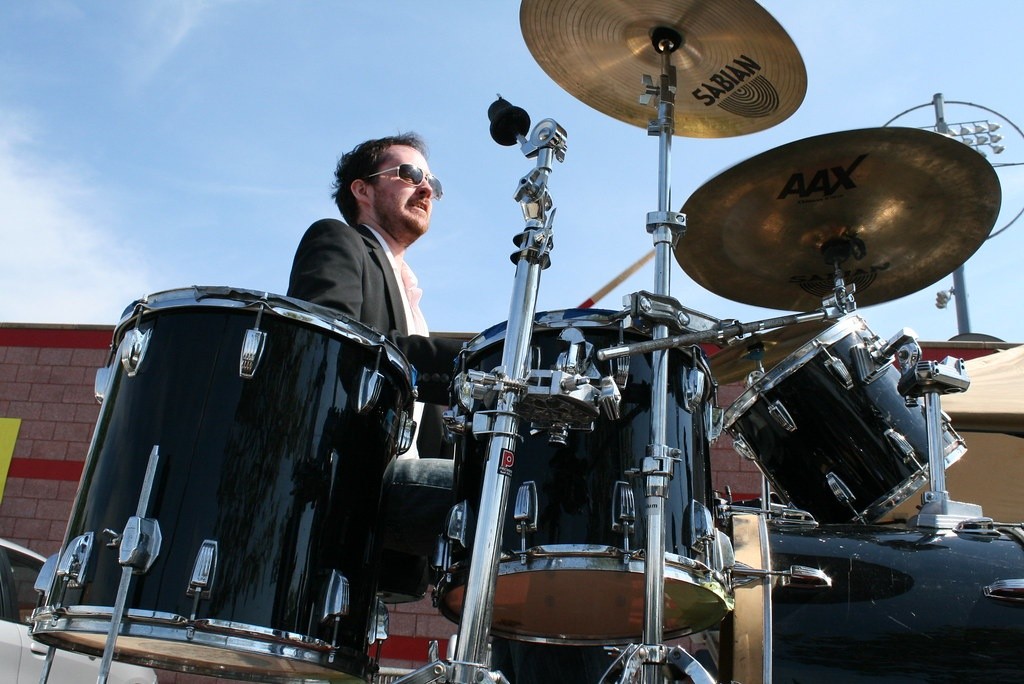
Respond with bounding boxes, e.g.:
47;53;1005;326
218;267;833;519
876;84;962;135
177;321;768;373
0;538;157;684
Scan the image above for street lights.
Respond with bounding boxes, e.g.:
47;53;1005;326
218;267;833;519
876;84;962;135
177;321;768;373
913;120;1008;334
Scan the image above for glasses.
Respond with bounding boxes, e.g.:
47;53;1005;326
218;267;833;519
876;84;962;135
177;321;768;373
366;164;444;201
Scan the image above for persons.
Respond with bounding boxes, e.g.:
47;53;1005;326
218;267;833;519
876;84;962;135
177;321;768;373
286;131;609;683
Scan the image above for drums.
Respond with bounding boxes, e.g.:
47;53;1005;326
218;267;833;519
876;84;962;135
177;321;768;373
726;507;1024;684
720;312;971;525
717;502;1023;684
426;305;740;651
455;627;626;684
24;279;424;684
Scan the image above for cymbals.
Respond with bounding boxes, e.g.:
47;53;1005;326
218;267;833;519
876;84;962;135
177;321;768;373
708;318;837;388
672;124;1005;318
517;0;809;140
